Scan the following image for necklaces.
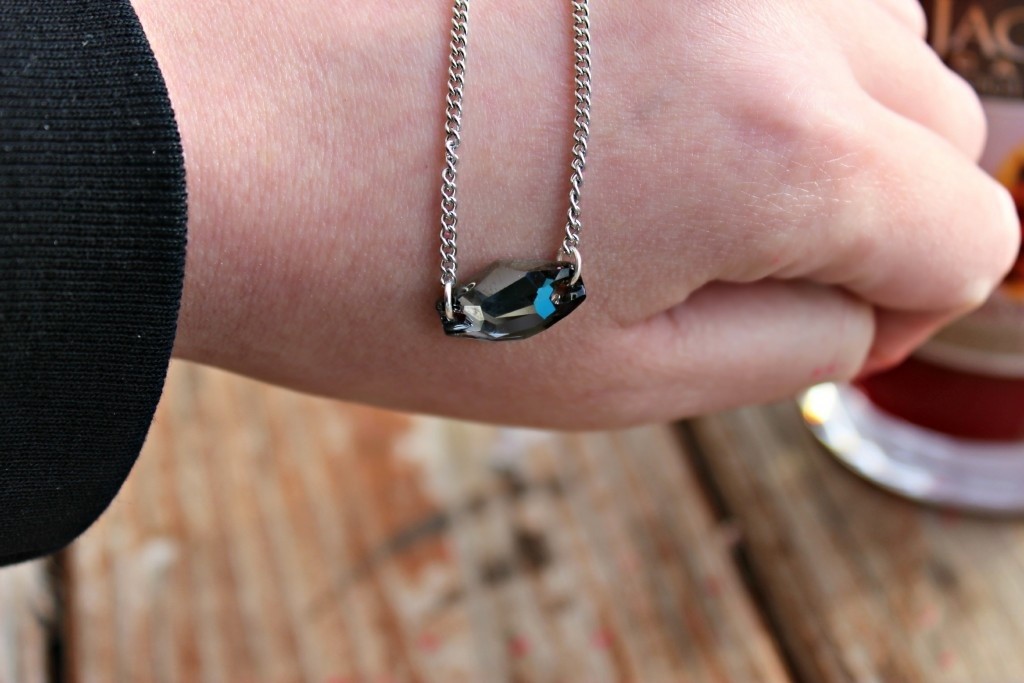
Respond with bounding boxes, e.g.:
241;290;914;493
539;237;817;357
437;0;594;342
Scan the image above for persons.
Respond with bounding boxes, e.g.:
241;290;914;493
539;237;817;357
0;0;1021;570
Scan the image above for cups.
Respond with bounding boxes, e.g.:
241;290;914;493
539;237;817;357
795;73;1024;520
